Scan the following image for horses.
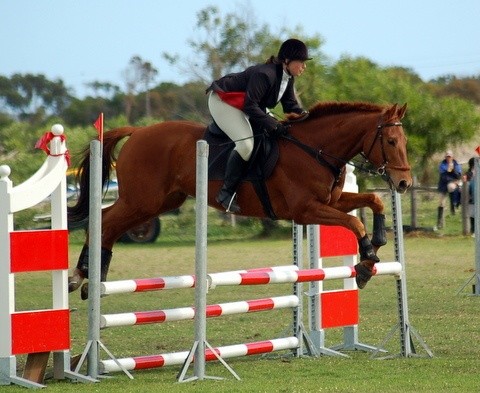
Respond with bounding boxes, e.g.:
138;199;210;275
50;100;412;301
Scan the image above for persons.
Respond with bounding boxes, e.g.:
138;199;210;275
207;38;313;212
436;149;477;235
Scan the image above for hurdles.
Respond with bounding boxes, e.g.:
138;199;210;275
174;138;434;384
0;123;388;393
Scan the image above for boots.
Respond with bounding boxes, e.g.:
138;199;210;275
437;207;444;229
216;150;248;212
449;191;456;215
470;216;475;233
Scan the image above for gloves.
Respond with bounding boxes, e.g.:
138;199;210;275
272;124;287;139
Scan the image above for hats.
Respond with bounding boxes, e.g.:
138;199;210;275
444;150;453;157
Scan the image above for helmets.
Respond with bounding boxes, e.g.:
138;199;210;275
277;39;312;62
